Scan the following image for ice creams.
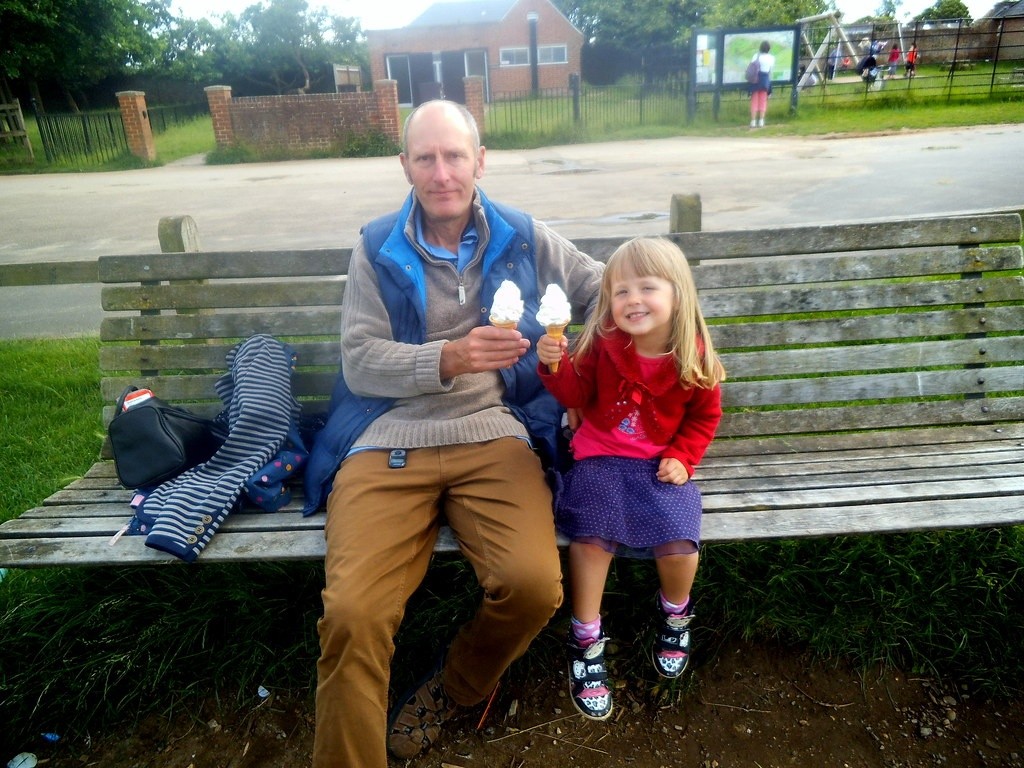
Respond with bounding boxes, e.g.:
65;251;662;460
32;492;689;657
536;284;572;373
488;279;524;369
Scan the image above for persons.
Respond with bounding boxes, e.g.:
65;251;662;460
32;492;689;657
305;99;609;768
903;42;917;77
747;41;773;127
888;43;901;77
840;56;850;74
827;46;841;80
535;234;723;720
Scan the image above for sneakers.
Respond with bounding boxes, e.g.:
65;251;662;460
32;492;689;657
386;656;459;762
564;624;613;722
651;589;696;680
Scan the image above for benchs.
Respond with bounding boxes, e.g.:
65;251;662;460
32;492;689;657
1;193;1024;565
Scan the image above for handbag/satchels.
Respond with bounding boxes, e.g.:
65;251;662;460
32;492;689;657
107;385;214;490
745;54;760;83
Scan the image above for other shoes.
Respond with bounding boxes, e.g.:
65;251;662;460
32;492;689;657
750;120;755;128
758;120;764;127
